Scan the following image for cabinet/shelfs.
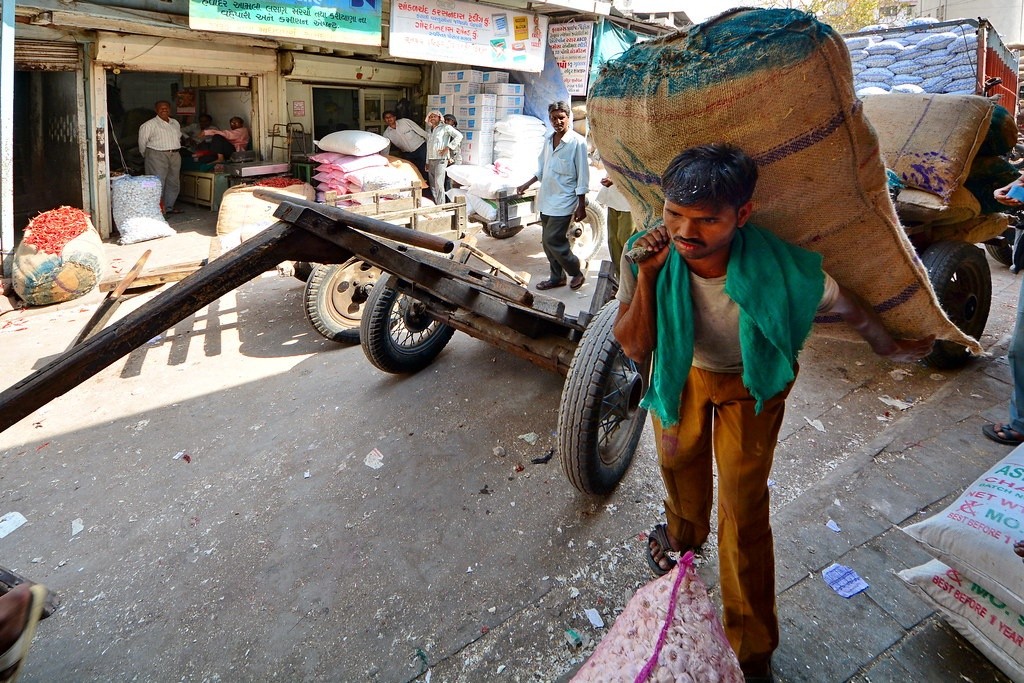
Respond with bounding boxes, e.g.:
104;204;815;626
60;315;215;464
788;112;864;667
178;170;228;212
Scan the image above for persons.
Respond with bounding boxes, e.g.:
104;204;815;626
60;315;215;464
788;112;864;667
595;172;638;298
517;101;589;290
612;144;936;683
982;174;1024;447
381;110;463;205
180;114;249;164
139;100;184;218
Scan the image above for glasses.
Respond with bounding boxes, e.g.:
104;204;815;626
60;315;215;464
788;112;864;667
230;122;241;125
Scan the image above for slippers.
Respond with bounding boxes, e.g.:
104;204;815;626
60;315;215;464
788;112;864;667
0;584;49;683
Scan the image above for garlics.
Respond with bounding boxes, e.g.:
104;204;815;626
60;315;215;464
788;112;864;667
567;566;746;683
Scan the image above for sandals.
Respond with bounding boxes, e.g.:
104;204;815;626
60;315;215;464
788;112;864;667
570;271;585;290
982;424;1024;446
536;275;567;290
740;658;774;683
647;524;701;576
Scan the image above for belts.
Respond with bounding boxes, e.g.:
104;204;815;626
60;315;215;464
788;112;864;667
146;147;179;152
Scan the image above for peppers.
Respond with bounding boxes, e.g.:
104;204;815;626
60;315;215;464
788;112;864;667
21;205;92;256
251;177;305;189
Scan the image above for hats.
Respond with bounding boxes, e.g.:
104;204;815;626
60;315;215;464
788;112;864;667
444;114;456;122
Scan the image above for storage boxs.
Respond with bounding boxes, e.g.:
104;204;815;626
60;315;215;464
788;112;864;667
425;70;525;165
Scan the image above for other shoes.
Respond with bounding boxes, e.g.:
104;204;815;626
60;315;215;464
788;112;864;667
166;207;184;213
163;214;168;221
